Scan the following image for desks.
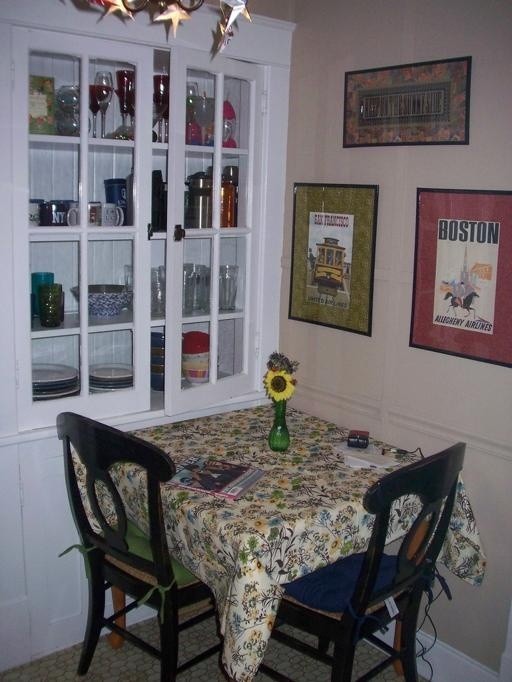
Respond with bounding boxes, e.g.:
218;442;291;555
69;401;486;680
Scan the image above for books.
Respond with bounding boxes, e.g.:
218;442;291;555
164;455;266;499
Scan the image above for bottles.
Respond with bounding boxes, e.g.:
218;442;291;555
187;164;238;230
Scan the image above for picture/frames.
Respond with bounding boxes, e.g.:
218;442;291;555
287;181;379;338
342;56;473;149
408;187;512;369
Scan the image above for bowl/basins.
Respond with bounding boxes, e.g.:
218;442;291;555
68;283;132;321
150;328;210;392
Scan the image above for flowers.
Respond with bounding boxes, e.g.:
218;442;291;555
264;350;298;403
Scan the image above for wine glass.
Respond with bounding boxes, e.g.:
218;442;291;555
59;67;235;147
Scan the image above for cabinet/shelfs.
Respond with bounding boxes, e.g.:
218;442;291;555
0;0;296;674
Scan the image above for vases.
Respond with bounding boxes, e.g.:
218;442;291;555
269;399;292;452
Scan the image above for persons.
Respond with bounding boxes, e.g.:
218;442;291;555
183;459;250;495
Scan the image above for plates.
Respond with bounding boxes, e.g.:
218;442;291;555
29;359;137;402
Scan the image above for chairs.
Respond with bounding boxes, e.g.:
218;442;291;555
56;411;231;681
253;439;467;681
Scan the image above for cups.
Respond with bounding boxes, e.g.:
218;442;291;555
32;271;65;328
150;263;237;318
29;165;168;229
